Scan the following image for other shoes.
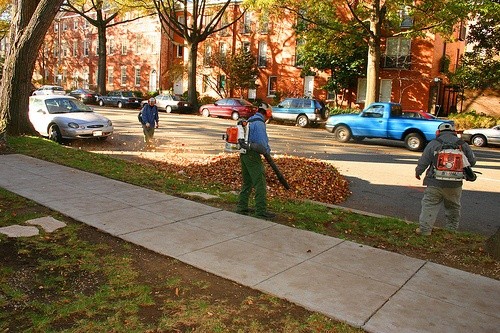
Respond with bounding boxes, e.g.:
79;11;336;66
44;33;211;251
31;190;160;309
416;228;431;235
239;208;254;214
256;212;276;219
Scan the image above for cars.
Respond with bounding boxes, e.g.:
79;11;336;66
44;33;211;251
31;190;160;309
140;94;192;113
199;97;259;120
31;85;67;96
248;99;272;124
462;124;500;147
69;89;102;104
96;91;146;108
401;110;437;120
29;95;115;144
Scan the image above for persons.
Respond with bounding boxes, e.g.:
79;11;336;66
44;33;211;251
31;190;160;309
415;122;476;236
141;97;159;143
236;103;276;218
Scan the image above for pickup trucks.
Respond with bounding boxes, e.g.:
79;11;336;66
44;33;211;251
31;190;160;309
325;102;455;152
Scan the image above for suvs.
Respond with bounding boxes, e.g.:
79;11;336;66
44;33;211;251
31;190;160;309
270;97;329;128
